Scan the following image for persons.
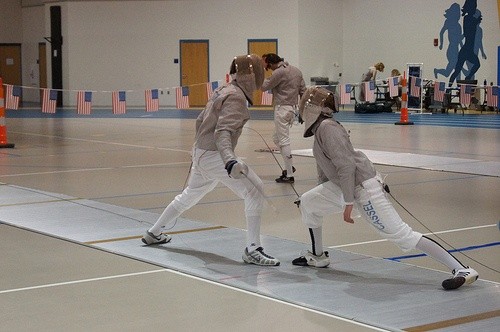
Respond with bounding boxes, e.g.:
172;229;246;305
260;54;307;184
141;55;281;266
369;63;384;80
292;87;479;290
392;69;400;76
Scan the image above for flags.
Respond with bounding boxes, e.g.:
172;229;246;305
176;86;189;108
389;77;398;97
77;90;92;115
42;89;57;113
487;85;498;106
340;85;351;104
145;89;159;112
207;81;218;98
365;82;374;101
461;84;471;104
411;76;421;97
262;89;273;105
434;81;445;102
6;86;20;110
112;90;126;114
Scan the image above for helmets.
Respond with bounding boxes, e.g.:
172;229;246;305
229;54;265;106
297;87;339;138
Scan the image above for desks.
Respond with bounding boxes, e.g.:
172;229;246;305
444;85;498;116
375;85;405;103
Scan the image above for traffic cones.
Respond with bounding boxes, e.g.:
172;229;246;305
395;78;414;125
0;77;15;148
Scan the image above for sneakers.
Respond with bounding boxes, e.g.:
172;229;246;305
283;167;296;175
292;251;330;267
142;230;172;244
275;176;294;183
442;266;479;289
242;246;280;266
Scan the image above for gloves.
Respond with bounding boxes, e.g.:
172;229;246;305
225;161;238;174
